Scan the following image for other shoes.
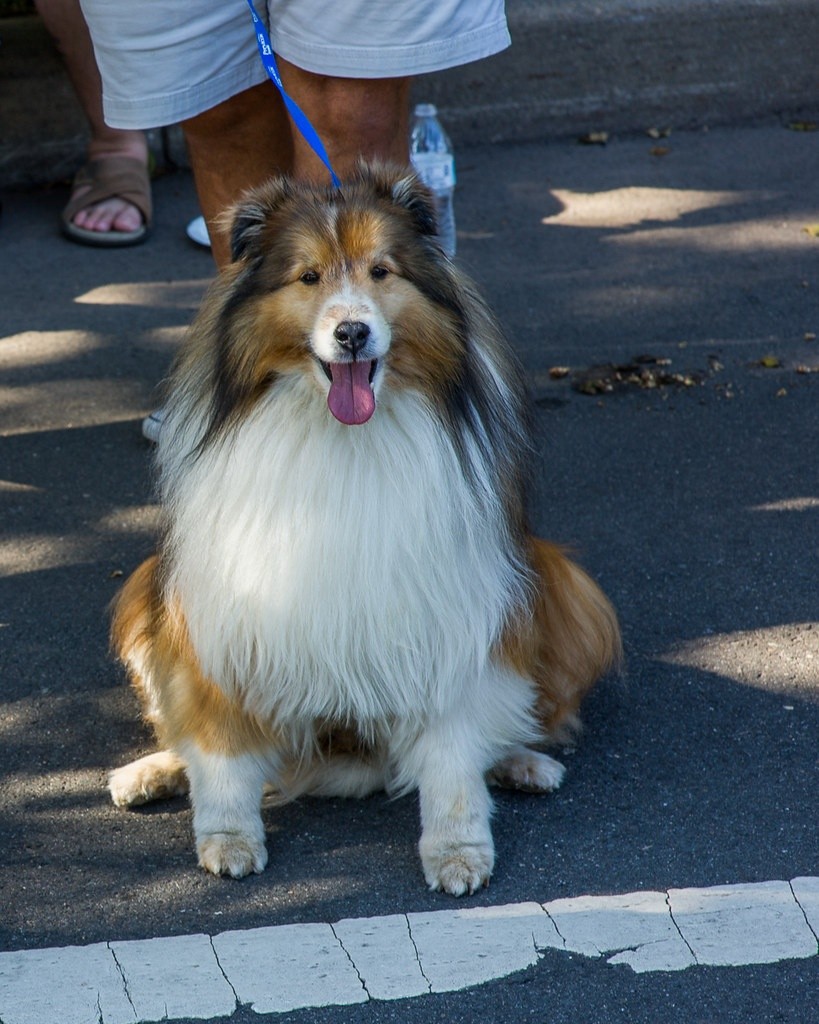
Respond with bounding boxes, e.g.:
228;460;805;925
141;408;166;440
186;215;210;247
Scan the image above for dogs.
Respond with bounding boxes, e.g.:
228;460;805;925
100;149;621;898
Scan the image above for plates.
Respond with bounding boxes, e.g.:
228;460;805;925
187;215;211;246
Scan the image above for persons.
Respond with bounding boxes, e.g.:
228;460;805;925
33;0;156;242
75;0;514;445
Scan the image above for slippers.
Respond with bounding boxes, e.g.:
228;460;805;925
63;156;153;246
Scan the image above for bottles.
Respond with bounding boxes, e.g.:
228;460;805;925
407;104;457;262
